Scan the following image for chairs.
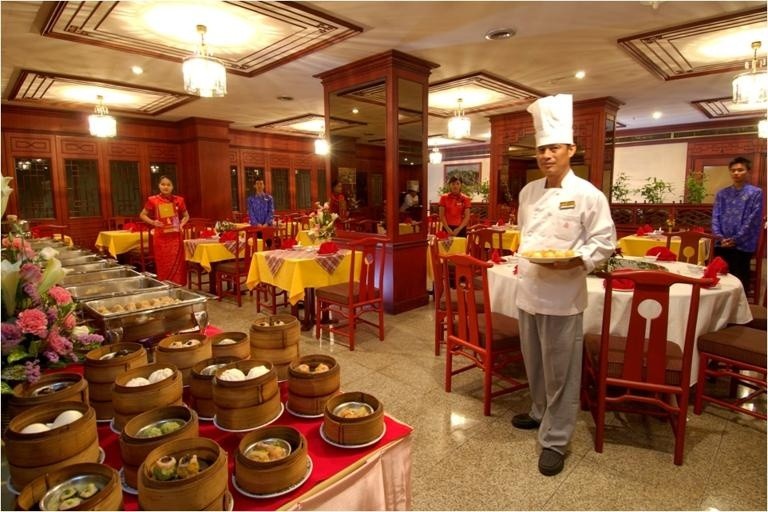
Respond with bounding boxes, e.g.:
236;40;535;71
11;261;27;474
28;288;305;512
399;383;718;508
691;328;766;426
96;211;767;352
428;234;489;357
578;267;708;465
436;251;530;419
31;223;67;241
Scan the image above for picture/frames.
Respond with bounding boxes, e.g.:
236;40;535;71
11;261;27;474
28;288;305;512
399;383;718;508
444;163;482;191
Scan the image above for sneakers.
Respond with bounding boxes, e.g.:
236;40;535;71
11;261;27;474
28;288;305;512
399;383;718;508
512;412;537;428
539;448;565;475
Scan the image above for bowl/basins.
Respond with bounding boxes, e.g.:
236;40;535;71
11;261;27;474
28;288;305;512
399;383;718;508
32;381;77;398
644;256;658;262
243;439;292;456
333;400;375;418
135;419;186;438
293;245;320;253
686;265;702;274
38;474;107;510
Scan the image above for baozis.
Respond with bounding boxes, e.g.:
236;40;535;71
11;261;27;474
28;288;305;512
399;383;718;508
19;316;370;510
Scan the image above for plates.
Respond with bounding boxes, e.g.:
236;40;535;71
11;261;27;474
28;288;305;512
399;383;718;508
196;416;213;421
96;419;113;423
232;454;313;498
6;446;105;495
319;421;388;449
285;390;344;418
119;466;139;495
213;400;284;433
515;248;582;265
109;416;121;436
225;490;234;512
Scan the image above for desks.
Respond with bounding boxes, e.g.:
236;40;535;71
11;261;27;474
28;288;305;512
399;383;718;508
1;326;413;511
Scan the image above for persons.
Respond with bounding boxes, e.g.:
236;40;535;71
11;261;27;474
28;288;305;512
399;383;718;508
248;179;274;246
711;157;762;298
330;180;350;230
140;174;189;288
439;176;472;288
511;91;616;476
400;190;420;212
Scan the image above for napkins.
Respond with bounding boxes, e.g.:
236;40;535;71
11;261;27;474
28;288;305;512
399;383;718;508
512;265;520;275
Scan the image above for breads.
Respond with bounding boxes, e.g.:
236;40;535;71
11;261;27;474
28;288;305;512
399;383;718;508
98;296;181;317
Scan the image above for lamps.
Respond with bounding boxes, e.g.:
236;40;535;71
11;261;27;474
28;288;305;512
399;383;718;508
182;25;227;97
315;127;327;155
733;41;768;113
429;145;442;164
448;98;470;139
88;95;116;138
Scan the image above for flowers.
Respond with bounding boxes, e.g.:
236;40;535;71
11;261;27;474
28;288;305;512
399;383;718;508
1;173;105;397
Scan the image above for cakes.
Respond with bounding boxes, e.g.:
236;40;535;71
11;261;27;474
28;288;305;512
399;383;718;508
521;248;578;261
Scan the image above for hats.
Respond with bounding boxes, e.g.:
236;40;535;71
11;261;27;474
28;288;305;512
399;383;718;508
407;183;419;192
527;94;574;148
